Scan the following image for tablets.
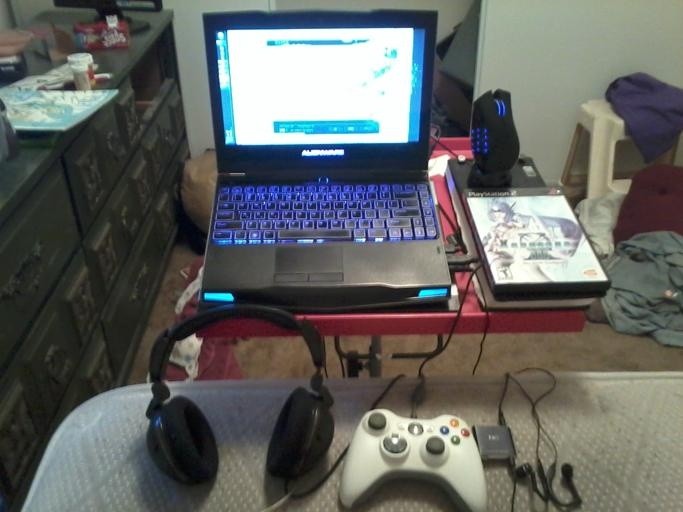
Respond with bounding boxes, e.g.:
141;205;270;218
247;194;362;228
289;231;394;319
461;186;611;295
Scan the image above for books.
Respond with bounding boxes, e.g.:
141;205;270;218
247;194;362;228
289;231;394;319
444;157;602;313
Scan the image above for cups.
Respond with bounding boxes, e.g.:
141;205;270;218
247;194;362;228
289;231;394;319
67;52;97;90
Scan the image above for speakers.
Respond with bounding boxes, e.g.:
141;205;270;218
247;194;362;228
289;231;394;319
469;86;520;190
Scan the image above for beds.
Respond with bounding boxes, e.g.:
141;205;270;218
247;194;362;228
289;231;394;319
21;368;683;512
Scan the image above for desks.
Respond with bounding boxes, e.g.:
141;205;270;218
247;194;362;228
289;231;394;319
196;137;586;378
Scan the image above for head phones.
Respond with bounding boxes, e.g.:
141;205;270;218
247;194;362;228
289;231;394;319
145;301;334;482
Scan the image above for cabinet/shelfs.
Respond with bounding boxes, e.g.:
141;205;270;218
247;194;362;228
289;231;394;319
0;10;192;512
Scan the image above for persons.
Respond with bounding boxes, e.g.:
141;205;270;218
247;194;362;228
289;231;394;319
485;195;577;262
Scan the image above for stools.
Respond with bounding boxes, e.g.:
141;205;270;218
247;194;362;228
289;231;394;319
560;98;683;199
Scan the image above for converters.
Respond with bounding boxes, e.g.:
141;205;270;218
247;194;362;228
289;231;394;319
473;423;516;462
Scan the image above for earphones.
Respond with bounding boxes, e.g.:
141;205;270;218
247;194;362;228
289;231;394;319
517;464;537;491
562;463;579;498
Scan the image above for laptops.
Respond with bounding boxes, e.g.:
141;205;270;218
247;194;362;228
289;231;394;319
200;7;453;315
